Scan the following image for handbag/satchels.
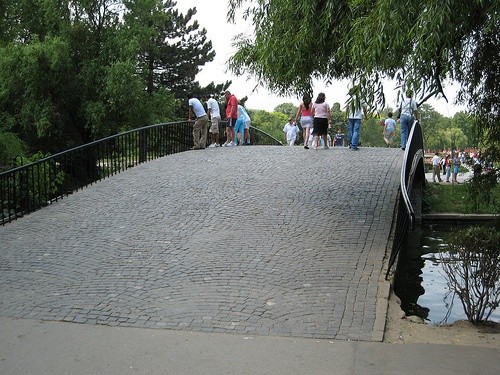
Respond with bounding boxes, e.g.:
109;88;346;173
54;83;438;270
397;111;401;118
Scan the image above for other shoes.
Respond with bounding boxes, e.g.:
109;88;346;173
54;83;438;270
387;144;390;148
401;147;405;150
313;146;318;150
348;145;359;151
324;146;329;149
234;142;250;146
433;180;457;183
304;145;309;149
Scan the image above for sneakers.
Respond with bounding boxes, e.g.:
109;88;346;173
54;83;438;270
207;144;216;147
199;146;205;149
227;141;234;146
214;143;220;146
222;140;230;146
190;146;199;150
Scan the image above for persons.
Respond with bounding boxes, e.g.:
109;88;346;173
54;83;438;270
347;86;368;150
187;92;208;150
383;113;396;147
397;89;417;150
283;117;300;146
440;156;447;175
335;128;345;147
238;103;252;145
431;153;443;183
233;104;246;146
311;93;331;149
445;154;451;182
222;91;238;147
294;93;315;149
333;137;337;147
454;146;486;171
205;94;221;148
453;153;461;182
310;126;332;148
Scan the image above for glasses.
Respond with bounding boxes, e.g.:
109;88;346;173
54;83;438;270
288;120;291;121
